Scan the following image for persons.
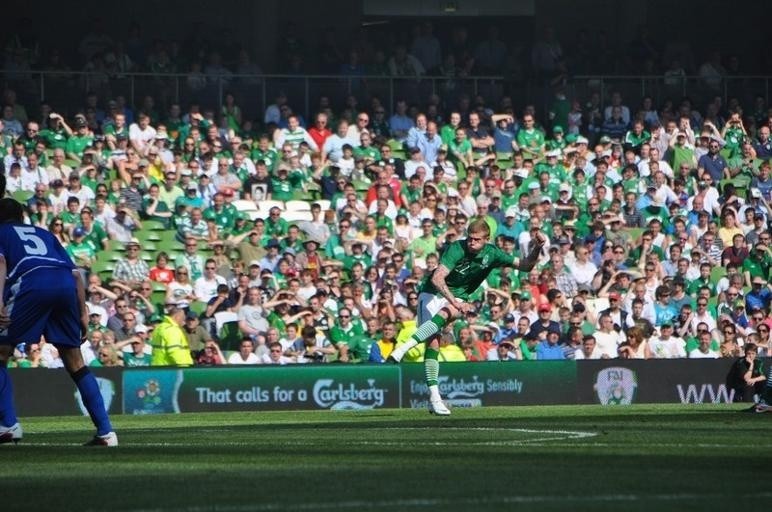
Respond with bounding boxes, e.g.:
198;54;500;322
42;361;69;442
0;198;119;447
726;343;772;413
0;93;772;370
388;219;546;415
0;19;739;89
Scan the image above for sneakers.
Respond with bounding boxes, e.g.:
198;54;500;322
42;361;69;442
81;431;119;447
429;399;451;416
0;422;23;445
389;345;408;365
755;402;770;414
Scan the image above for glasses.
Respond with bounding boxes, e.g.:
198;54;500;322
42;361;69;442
26;107;769;359
